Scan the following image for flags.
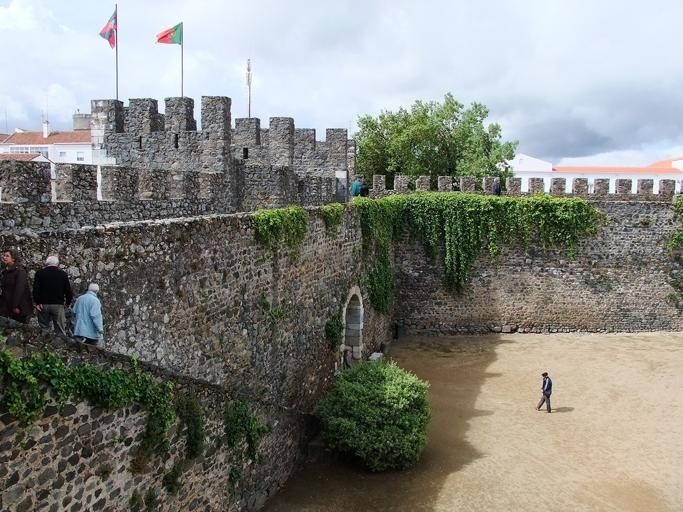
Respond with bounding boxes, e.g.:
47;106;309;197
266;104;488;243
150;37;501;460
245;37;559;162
154;21;183;46
98;3;118;50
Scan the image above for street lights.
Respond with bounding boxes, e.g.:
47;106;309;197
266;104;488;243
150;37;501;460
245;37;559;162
245;60;253;116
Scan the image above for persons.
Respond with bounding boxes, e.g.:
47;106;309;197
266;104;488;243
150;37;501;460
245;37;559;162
348;175;362;197
31;254;73;337
492;178;502;197
70;282;104;349
359;181;370;198
0;247;35;327
532;372;552;413
